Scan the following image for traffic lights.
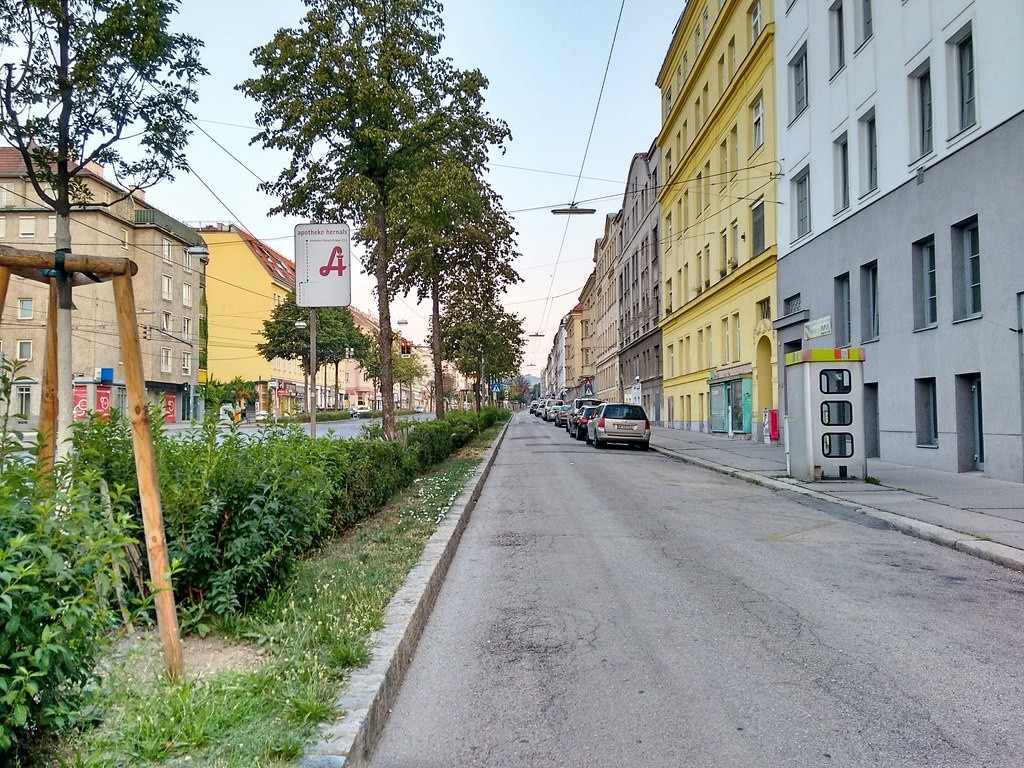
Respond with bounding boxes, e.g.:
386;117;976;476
143;325;150;340
472;383;476;396
183;382;188;391
400;342;412;354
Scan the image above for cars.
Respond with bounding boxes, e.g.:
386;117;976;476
570;406;598;441
345;407;358;418
416;406;424;413
357;406;371;416
548;406;561;420
555;405;570;426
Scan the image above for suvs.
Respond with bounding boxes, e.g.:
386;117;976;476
587;403;651;452
529;399;564;421
566;397;606;431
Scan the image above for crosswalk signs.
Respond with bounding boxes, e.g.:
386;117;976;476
586;387;594;395
492;384;500;392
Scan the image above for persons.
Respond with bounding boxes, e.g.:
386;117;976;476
302;407;305;412
